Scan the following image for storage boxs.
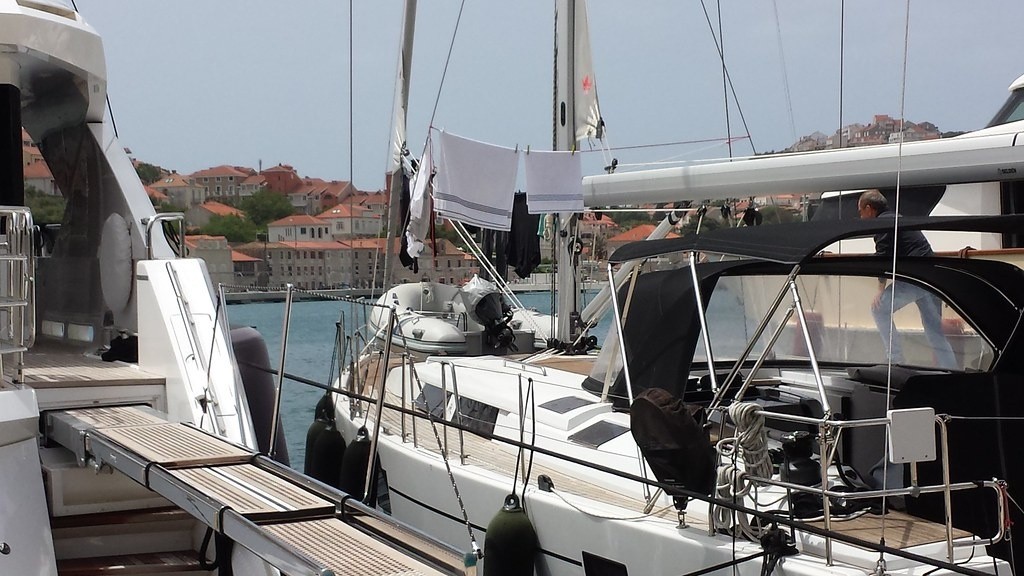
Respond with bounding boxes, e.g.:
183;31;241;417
464;330;535;356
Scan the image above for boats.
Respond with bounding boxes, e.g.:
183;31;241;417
761;76;1023;375
1;0;289;574
367;279;558;356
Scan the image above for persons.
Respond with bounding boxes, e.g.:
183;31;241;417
858;191;961;373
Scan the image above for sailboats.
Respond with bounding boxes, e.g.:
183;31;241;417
305;0;1023;576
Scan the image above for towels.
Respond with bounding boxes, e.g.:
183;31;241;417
431;128;522;233
523;149;586;215
